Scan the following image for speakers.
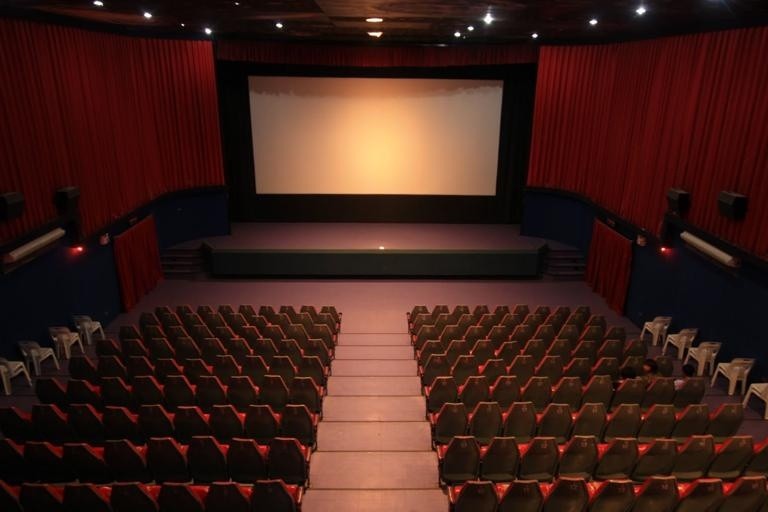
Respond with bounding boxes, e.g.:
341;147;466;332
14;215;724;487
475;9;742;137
0;191;27;223
663;188;691;215
53;185;81;210
717;190;748;220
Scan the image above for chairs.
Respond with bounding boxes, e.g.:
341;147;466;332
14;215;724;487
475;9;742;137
124;339;149;354
521;376;552;409
254;338;279;353
603;325;624;340
103;406;139;431
506;356;536;386
165;312;182;326
720;477;767;512
478;314;498;335
406;306;428;333
251;315;268;330
280;339;304;354
318;312;339;333
409;313;431;344
675;480;726;512
274;313;292;331
321;305;341;321
474;305;489;323
641;316;671;346
569;312;586;331
445;339;470;366
472;338;495;365
0;356;31;395
3;438;22;462
146;436;193;471
503;403;536;443
244;405;280;434
598;339;626;365
631;476;679;512
167;326;188;335
447;480;499;512
452;306;469;321
217;304;233;312
710;358;755;395
258;305;274;314
33;376;66;400
592;358;619;376
573;305;590;315
632;438;677;480
479;438;520;481
571;404;607;445
496;478;545;512
671;405;709;439
192;324;214;337
67;377;100;399
551;377;584;409
96;356;126;372
458;314;476;334
207;312;226;329
228;439;270;473
164;375;196;397
312;324;336;348
258;375;289;399
155;357;181;373
518;439;561;481
269;355;298;375
492;374;522;409
522;314;543;335
250;479;303;511
707;435;754;479
412;326;438;359
306;338;335;362
554;305;571;323
198;305;214;313
297;313;314;328
239;304;254;314
228;312;250;328
706;404;743;442
605;403;641;438
563;358;591;385
204;482;252;512
431;304;449;322
451;355;481;386
745;438;768;475
290;376;325;400
419;355;451;395
548;337;569;361
61;483;111;511
440;326;464;350
215;327;239;338
534;355;562;386
644;378;675;405
73;314;105;344
742;383;768;420
263;325;285;338
614;380;647;409
672;436;715;479
428;404;470;450
95;340;123;353
0;480;18;512
620;357;644;371
132;376;163;397
544;314;563;336
153;304;170;313
140;404;175;432
626;339;648;357
523;339;547;365
415;339;443;375
184;358;212;373
281;403;319;451
494;306;510;322
213;355;241;374
438;436;479;484
585;315;606;327
202;337;228;354
435;314;458;336
21;442;64;467
573;341;598;366
119;324;142;337
656;356;671;376
460;376;489;411
193;434;229;472
177;305;192;313
299;356;330;385
50;326;86;359
558;436;598;477
638;404;677;437
100;376;132;398
229;337;252;353
175;406;209;432
111;483;160;512
662;329;697;360
595;440;639;479
150;337;174;354
499;314;521;335
127;355;154;372
581;376;615;408
209;404;243;434
513;304;530;323
33;404;68;430
1;405;32;429
497;341;522;366
464;326;486;350
534;405;572;444
19;482;60;512
540;476;590;512
675;379;705;405
556;324;579;347
532;324;554;348
534;305;551;323
158;482;209;512
68;403;103;431
468;403;502;445
286;323;309;339
588;478;635;512
486;326;507;349
139;312;160;325
185;312;202;325
19;340;60;377
272;436;311;490
578;325;603;345
63;441;105;467
228;375;259;398
195;375;227;398
144;326;163;336
301;305;317;316
241;325;261;337
684;342;721;376
68;355;95;372
280;305;295;316
175;336;200;354
508;326;531;348
423;378;458;420
478;359;508;385
103;440;145;472
242;355;268;374
